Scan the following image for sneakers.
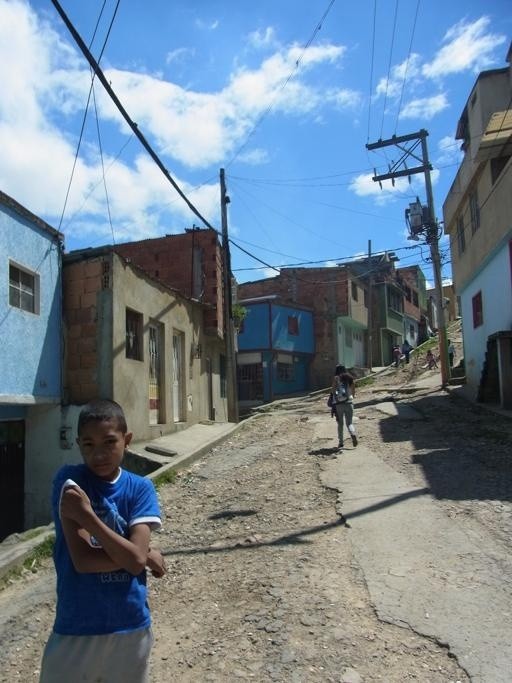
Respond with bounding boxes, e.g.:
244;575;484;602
336;435;360;447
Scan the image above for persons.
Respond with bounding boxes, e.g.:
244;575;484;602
328;364;357;447
426;350;438;370
402;340;411;363
393;345;402;366
39;398;167;683
448;340;457;367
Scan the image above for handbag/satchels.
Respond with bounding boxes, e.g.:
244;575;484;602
330;376;348;405
